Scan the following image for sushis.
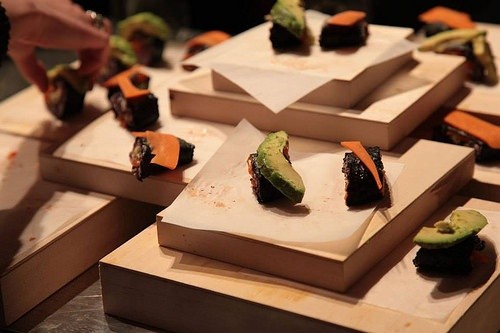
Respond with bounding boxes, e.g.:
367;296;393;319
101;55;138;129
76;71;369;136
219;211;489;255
246;130;306;206
414;209;490;277
434;110;500;165
415;9;498;84
128;130;196;183
319;9;370;50
42;12;233;134
266;0;317;54
340;140;393;209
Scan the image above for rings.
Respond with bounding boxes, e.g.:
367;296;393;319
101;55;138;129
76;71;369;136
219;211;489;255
86;10;104;32
